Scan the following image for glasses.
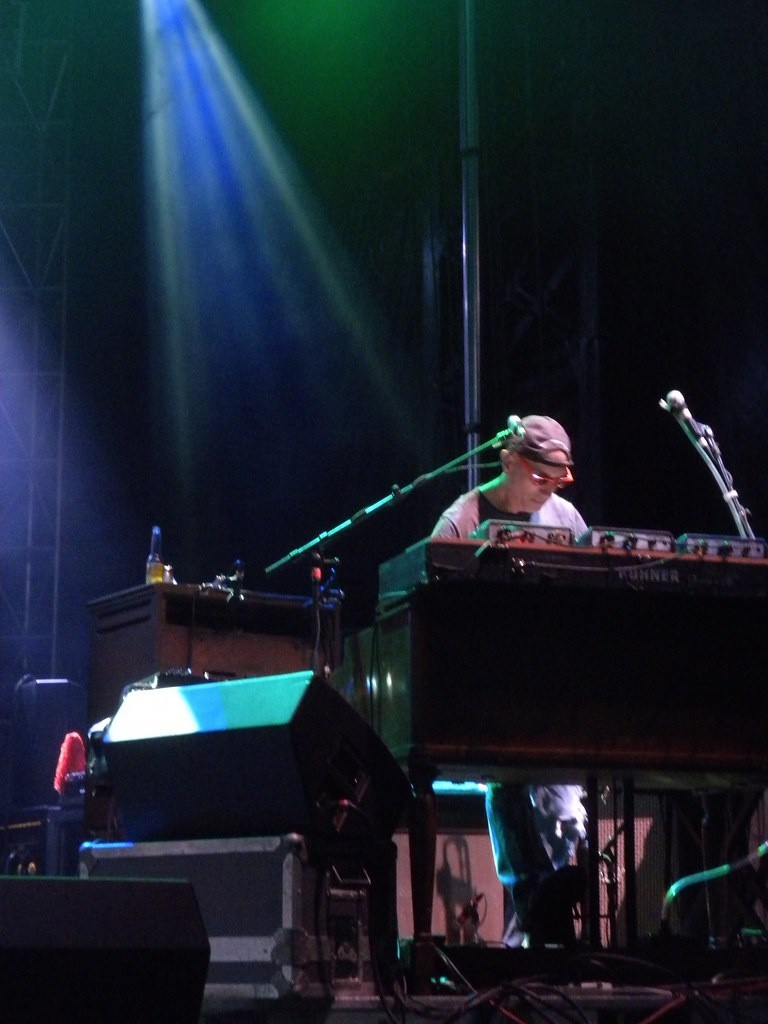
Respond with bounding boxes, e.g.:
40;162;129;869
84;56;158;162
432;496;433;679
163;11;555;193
513;449;574;489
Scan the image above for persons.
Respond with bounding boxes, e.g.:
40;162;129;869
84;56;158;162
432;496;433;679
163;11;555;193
433;415;588;949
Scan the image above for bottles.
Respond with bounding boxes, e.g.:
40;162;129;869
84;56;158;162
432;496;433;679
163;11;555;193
145;526;164;584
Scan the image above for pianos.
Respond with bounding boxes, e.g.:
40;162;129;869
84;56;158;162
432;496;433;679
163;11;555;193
344;595;765;779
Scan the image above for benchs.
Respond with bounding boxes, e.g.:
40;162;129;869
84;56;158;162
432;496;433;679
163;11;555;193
395;780;488;835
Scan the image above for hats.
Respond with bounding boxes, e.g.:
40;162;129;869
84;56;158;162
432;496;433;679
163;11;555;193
507;415;574;466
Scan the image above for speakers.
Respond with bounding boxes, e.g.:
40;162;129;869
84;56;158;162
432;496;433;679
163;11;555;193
101;669;414;878
0;874;211;1024
569;788;672;954
392;825;504;943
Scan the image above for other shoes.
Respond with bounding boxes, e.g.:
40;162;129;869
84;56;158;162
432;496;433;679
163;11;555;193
530;866;586;942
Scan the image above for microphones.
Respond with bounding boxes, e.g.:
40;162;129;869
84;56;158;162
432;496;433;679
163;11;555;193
666;389;711;454
508;415;526;437
456;894;482;928
